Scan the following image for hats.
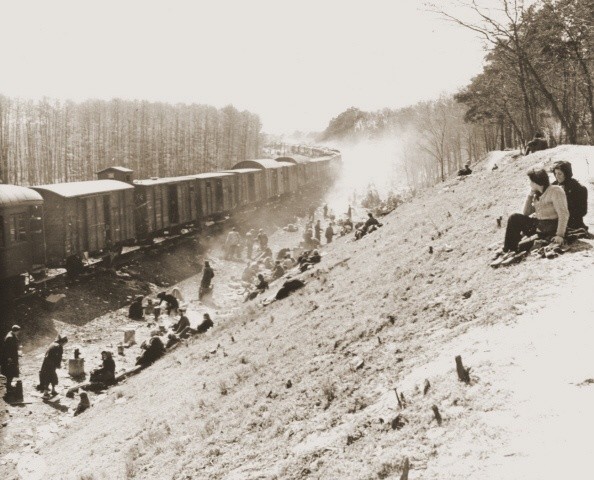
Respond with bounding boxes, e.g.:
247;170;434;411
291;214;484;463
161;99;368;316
528;167;547;182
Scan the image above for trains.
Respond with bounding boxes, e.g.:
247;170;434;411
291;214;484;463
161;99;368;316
0;146;340;296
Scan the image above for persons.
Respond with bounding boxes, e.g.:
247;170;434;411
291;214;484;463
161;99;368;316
1;325;23;391
241;261;257;284
269;259;284;279
172;310;191;335
302;220;323;251
344;204;354;218
550;160;588;242
307;204;317;220
157;291;179;317
324;204;328;218
226;227;243;261
245;229;258;259
456;164;472;176
365;213;381;236
86;350;116;382
178;313;215;340
341;218;353;236
143;297;159;316
254;245;273;263
128;294;144;321
251;276;271;298
492;166;570;267
258;228;269;252
39;334;68;398
325;221;337;244
60;391;66;399
198;259;216;289
523;133;548;156
